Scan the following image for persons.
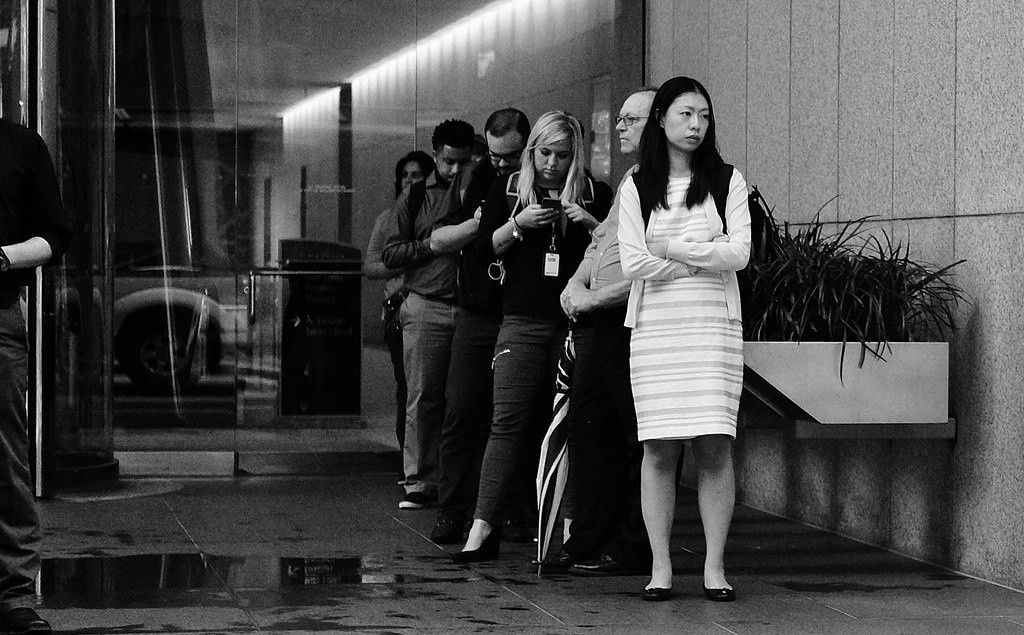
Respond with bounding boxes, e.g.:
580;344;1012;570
618;75;750;601
0;119;69;635
530;85;680;575
449;111;615;564
363;107;536;543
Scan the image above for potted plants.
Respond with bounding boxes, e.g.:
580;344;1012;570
739;184;975;425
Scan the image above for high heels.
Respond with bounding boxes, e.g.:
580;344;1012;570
451;528;501;562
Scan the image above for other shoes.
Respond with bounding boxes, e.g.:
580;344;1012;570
431;513;467;543
398;472;408;485
497;519;534;542
399;492;437;508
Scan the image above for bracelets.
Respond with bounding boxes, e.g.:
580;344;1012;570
509;217;523;235
508;219;523;242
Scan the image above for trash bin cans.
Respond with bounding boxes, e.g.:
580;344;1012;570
276;241;362;414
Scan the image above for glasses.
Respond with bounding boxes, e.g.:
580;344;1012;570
485;150;522;162
615;115;648;126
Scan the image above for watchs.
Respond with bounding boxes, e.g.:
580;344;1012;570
0;247;10;273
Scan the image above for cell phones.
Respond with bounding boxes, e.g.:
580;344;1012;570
542;198;561;223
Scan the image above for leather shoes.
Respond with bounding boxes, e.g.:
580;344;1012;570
569;549;625;576
702;582;736;601
2;606;52;634
528;544;572;573
642;578;672;600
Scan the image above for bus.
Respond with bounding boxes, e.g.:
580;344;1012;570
45;109;286;390
